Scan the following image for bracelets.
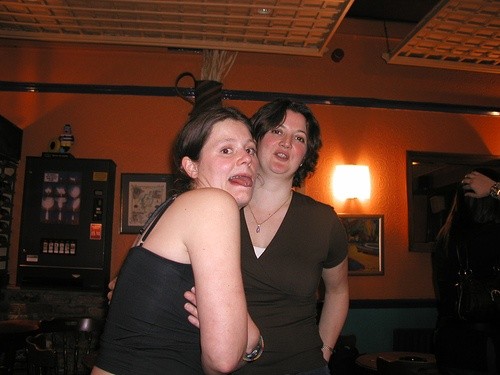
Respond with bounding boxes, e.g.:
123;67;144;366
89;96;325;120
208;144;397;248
323;345;333;355
241;334;265;362
490;182;500;200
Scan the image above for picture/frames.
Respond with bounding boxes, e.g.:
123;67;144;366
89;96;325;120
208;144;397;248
339;212;385;276
119;172;175;234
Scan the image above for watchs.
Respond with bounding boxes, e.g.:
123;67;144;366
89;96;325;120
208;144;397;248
243;335;262;359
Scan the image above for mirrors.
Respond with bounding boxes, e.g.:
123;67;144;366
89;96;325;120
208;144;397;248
406;150;500;253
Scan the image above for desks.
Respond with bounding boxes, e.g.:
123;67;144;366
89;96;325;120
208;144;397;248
356;352;436;371
0;320;38;375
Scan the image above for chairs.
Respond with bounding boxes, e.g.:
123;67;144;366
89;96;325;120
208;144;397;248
328;321;492;375
26;317;107;375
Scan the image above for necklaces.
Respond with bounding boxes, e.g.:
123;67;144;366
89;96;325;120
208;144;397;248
247;188;292;233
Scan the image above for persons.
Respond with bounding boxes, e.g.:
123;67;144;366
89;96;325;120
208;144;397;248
107;97;349;375
431;158;500;375
90;106;260;375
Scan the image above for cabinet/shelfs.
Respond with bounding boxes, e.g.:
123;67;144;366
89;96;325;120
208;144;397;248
16;156;116;292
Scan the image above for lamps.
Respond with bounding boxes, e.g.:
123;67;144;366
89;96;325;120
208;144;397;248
332;164;371;202
